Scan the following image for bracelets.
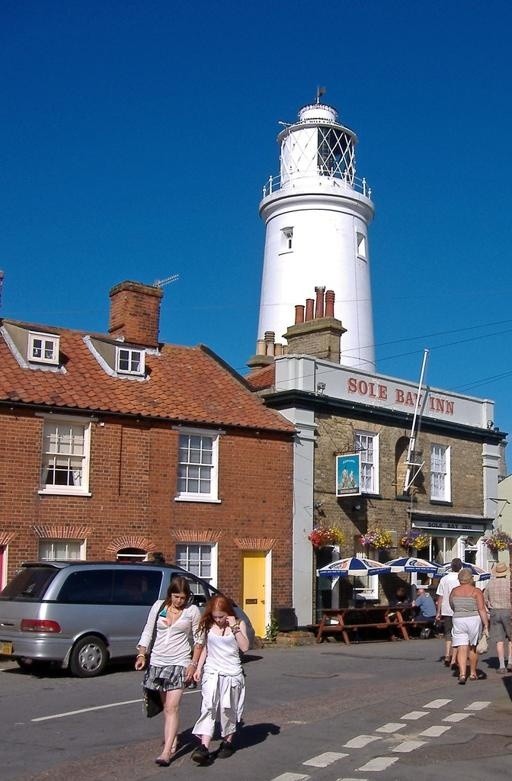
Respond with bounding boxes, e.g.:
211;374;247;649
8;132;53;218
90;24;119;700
136;654;145;659
229;624;241;635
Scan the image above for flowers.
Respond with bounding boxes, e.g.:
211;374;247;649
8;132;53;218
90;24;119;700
307;526;512;552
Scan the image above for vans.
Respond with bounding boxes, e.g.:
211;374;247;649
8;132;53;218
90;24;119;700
0;557;255;680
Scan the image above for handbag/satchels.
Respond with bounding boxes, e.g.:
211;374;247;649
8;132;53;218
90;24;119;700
144;671;163;718
477;629;489;653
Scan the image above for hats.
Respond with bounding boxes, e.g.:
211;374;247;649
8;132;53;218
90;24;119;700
492;562;510;577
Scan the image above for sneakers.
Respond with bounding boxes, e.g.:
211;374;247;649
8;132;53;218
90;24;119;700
155;739;235;765
506;663;512;671
496;668;505;674
444;656;451;664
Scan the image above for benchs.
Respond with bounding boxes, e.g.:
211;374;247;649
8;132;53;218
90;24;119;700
332;620;420;640
306;624;322;632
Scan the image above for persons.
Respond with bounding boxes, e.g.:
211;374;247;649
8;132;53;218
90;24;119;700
341;466;355;489
314;555;512;686
134;576;206;767
191;596;250;767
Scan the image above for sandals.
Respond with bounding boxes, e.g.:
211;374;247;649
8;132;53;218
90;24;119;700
470;674;479;680
459;676;465;685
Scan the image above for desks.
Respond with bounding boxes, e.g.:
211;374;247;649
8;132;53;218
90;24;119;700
305;600;423;645
